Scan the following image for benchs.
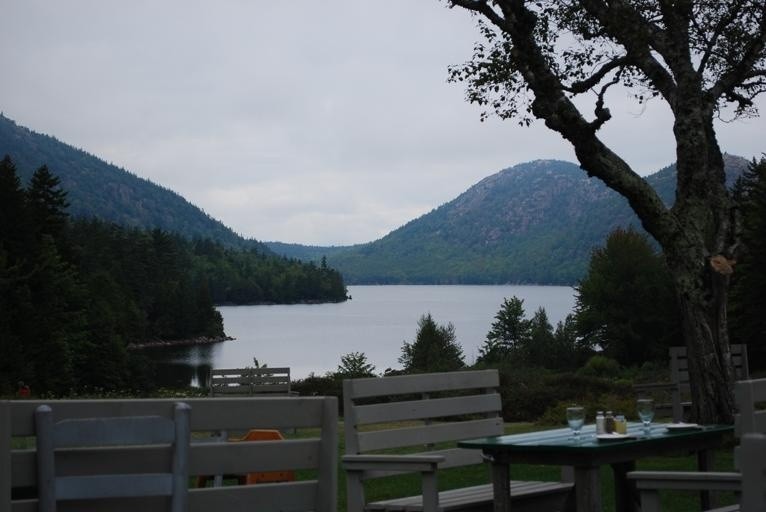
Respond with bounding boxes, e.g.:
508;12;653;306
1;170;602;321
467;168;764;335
635;344;749;422
0;399;336;512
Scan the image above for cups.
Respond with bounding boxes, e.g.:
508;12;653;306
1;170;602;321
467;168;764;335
613;418;627;435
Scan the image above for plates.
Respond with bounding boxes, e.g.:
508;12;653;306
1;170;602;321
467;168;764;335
595;434;629;441
665;422;699;431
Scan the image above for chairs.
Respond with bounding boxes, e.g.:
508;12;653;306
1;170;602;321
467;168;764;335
205;368;299;403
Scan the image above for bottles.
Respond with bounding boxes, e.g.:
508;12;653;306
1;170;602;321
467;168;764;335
595;411;605;435
605;410;615;434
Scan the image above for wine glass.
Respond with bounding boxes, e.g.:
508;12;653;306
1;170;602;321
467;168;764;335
635;398;657;438
566;406;587;441
